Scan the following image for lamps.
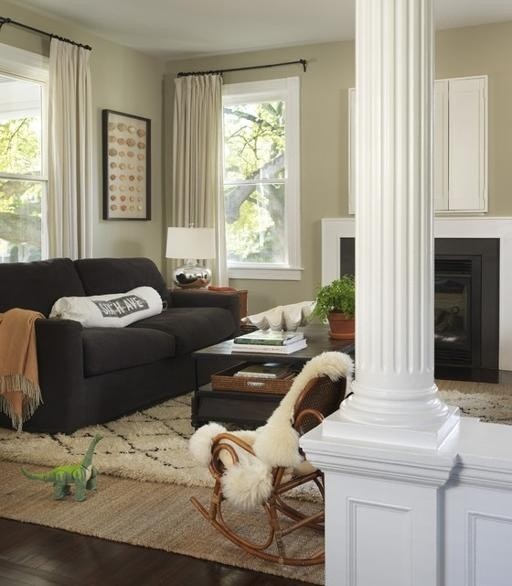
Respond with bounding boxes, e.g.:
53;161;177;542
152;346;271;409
165;226;217;289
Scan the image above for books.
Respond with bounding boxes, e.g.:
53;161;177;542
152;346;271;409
233;371;293;377
233;328;305;345
232;338;309;354
238;363;289;376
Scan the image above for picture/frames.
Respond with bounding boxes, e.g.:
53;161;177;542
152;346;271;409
101;108;152;221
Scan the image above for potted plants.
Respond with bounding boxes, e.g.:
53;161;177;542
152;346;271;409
303;273;354;340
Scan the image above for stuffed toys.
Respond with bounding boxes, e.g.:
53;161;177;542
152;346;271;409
18;433;104;501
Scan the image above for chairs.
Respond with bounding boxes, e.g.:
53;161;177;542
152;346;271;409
187;350;355;566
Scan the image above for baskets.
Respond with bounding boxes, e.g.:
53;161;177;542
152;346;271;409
209;360;294;395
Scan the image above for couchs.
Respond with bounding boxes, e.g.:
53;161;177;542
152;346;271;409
1;256;243;434
